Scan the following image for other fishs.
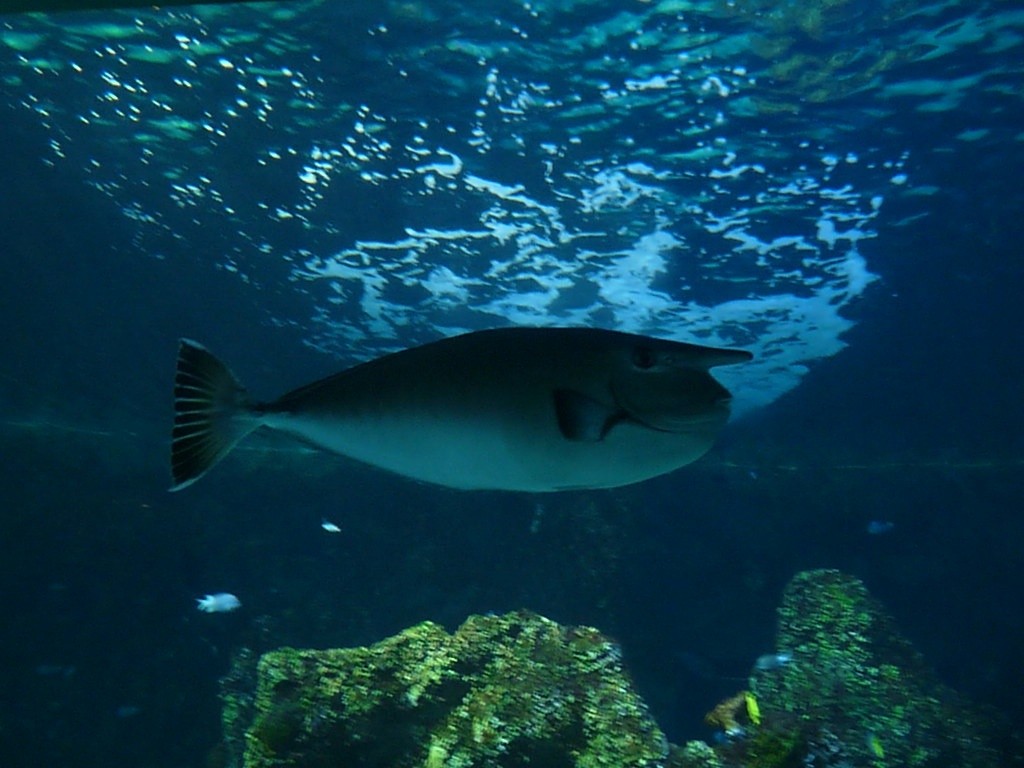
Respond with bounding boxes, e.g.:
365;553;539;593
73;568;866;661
195;592;241;614
165;323;754;495
